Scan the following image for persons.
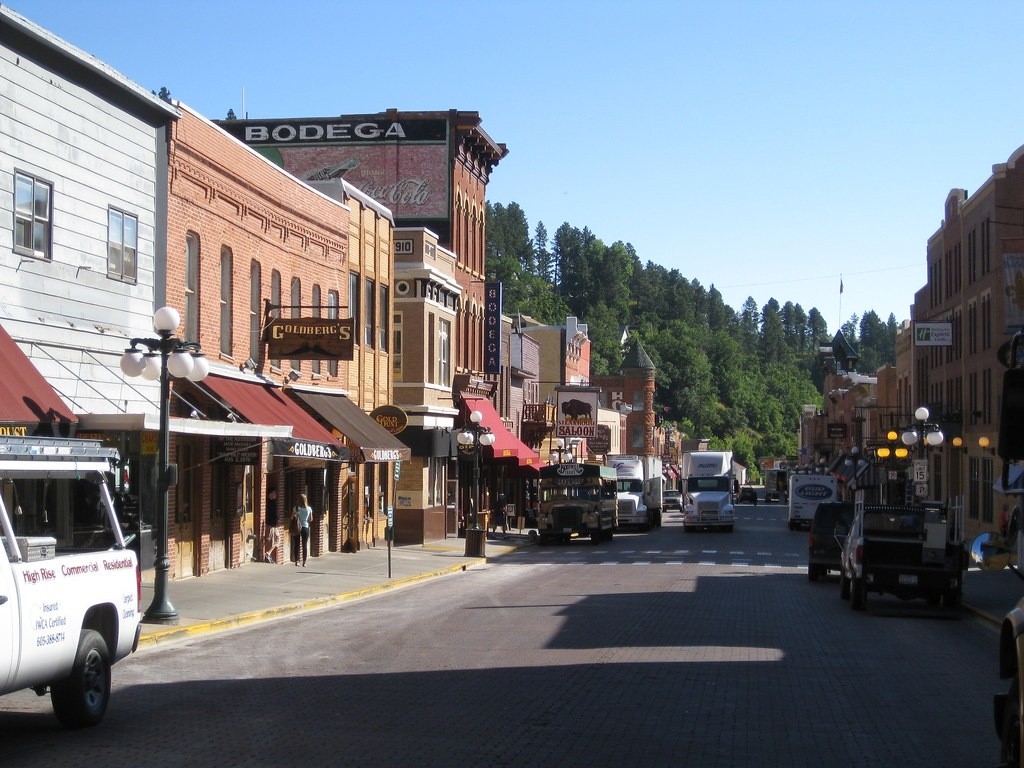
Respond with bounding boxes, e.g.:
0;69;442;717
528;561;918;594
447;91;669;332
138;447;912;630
288;494;312;568
998;503;1011;539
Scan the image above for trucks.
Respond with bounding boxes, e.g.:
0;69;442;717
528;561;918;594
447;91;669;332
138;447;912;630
602;453;663;532
787;474;840;530
764;469;789;502
682;450;739;533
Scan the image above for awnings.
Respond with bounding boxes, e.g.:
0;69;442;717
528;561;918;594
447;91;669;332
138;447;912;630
992;464;1024;496
464;396;548;471
1;324;79;438
199;373;411;463
826;455;864;482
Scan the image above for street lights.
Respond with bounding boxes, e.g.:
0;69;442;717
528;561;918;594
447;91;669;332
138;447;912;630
120;305;210;627
901;407;944;510
844;447;866;490
457;410;496;556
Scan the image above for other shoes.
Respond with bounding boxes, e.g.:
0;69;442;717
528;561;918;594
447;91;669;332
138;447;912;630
504;535;507;539
296;561;300;566
265;551;275;564
491;532;496;538
303;563;306;566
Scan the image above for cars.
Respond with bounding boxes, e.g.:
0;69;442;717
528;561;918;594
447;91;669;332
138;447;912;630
807;501;970;619
662;490;683;512
738;486;757;504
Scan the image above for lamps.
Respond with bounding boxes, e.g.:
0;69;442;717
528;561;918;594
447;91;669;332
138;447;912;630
191;411;200;419
952;437;967;454
227;413;237;423
924;438;943;453
444;426;451;433
978;437;995;456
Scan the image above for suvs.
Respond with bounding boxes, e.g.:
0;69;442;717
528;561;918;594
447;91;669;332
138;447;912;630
0;433;143;730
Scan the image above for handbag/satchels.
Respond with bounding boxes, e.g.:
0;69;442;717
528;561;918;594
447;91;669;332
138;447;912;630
289;505;302;536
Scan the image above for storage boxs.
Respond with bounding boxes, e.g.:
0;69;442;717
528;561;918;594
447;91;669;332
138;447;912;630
1;537;57;563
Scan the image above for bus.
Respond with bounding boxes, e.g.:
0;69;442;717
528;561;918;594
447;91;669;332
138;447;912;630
539;463;618;545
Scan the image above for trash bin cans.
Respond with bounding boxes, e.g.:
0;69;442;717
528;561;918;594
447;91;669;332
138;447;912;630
463;529;487;557
